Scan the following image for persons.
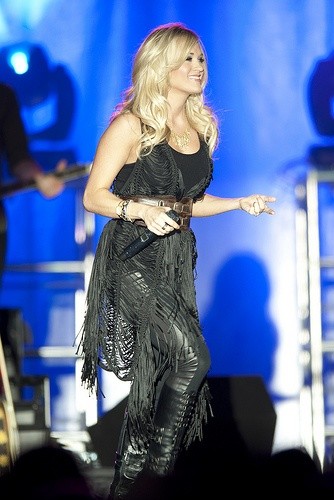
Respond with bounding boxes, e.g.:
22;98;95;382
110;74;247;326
0;82;67;278
83;22;277;499
0;441;334;500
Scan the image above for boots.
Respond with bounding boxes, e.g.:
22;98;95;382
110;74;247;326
109;405;151;500
143;385;197;476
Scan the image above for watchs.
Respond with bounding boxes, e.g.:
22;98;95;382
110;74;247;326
115;199;135;222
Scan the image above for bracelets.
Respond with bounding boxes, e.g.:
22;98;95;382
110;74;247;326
239;198;243;210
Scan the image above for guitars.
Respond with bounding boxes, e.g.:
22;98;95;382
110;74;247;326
0;162;93;226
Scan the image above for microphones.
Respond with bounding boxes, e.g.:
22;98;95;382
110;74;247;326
119;210;179;262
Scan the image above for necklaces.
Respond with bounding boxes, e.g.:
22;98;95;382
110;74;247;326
169;126;191;147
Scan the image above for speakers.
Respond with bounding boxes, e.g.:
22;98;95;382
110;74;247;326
87;377;277;471
